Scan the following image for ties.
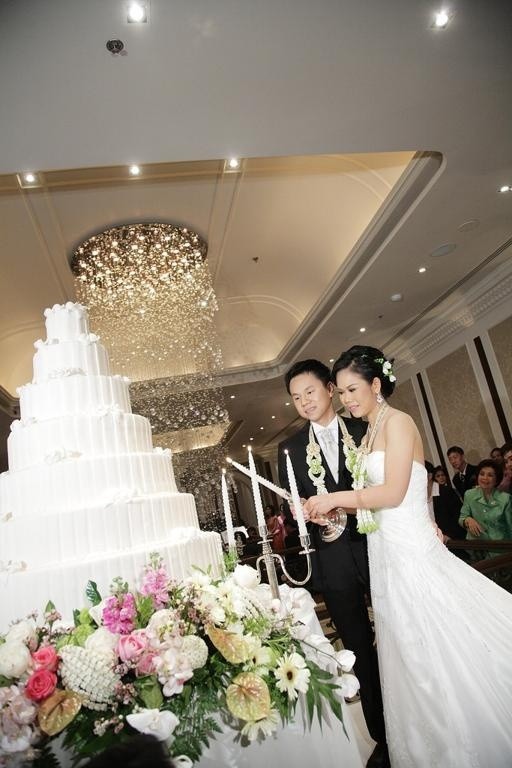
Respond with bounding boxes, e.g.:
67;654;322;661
323;430;338;479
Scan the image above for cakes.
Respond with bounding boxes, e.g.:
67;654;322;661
0;301;230;639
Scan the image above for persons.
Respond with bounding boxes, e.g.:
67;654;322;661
234;503;302;564
279;346;512;768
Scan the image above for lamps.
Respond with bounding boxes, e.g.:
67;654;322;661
70;220;244;552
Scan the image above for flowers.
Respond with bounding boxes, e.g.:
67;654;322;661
0;554;363;768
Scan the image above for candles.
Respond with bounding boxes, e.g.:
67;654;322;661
213;446;309;540
